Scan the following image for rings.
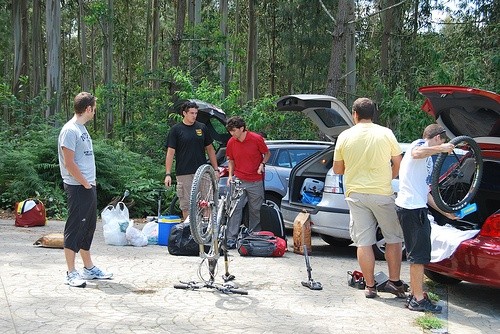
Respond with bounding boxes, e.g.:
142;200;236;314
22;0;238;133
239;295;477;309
452;145;454;147
448;146;450;148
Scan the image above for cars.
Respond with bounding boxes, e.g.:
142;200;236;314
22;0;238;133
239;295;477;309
419;85;500;292
275;94;411;260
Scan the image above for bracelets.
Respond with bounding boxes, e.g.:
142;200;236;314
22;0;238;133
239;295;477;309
215;169;218;171
261;162;265;167
166;173;170;176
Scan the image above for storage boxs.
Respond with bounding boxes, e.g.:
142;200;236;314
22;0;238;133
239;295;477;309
158;216;181;246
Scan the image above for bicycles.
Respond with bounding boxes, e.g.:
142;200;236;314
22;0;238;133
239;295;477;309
173;163;248;294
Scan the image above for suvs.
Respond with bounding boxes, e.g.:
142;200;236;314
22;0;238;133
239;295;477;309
216;139;335;207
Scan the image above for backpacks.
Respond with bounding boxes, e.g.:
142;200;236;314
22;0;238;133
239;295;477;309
237;224;285;257
260;200;284;238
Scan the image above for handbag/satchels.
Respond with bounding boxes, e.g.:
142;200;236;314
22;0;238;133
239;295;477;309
168;222;223;256
102;202;159;247
14;199;45;226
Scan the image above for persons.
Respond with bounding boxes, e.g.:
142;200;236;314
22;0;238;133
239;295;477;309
333;98;408;299
226;116;271;249
58;92;113;287
394;124;460;314
165;101;220;220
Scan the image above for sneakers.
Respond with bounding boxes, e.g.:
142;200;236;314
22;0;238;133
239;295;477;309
405;292;442;313
84;265;113;280
365;282;377;298
64;270;86;287
347;270;366;289
385;281;406;297
